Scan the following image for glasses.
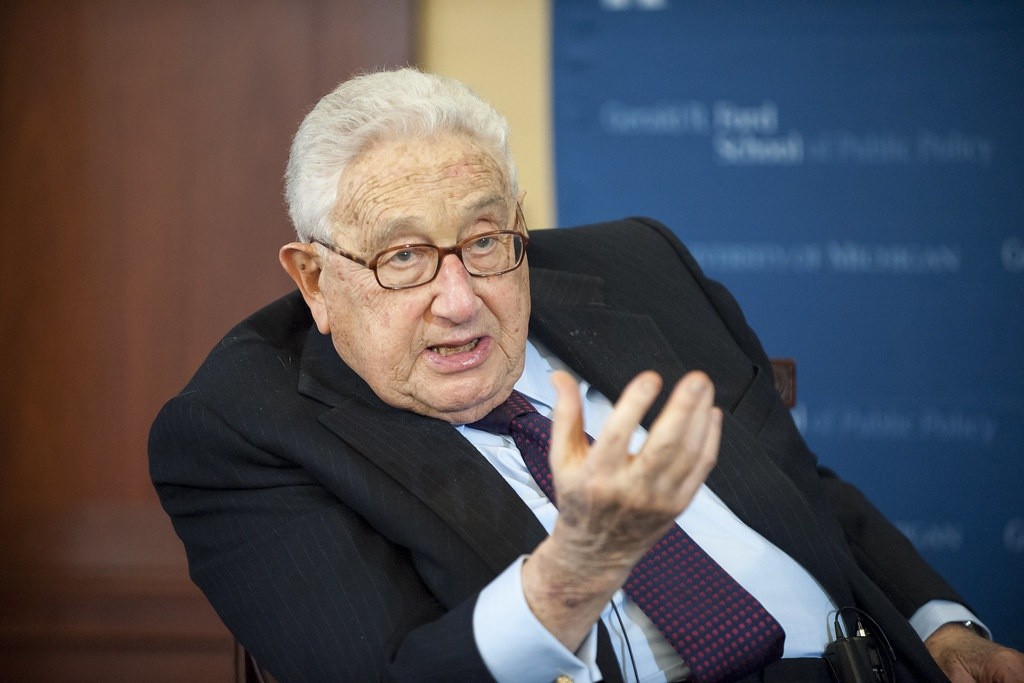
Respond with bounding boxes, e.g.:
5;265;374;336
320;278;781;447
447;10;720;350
303;200;529;292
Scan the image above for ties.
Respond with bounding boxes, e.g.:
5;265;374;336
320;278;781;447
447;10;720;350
464;389;787;683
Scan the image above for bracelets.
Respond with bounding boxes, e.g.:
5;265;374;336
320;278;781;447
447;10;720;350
954;618;980;632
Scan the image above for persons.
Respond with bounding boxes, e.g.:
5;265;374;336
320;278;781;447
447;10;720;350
143;64;1022;683
